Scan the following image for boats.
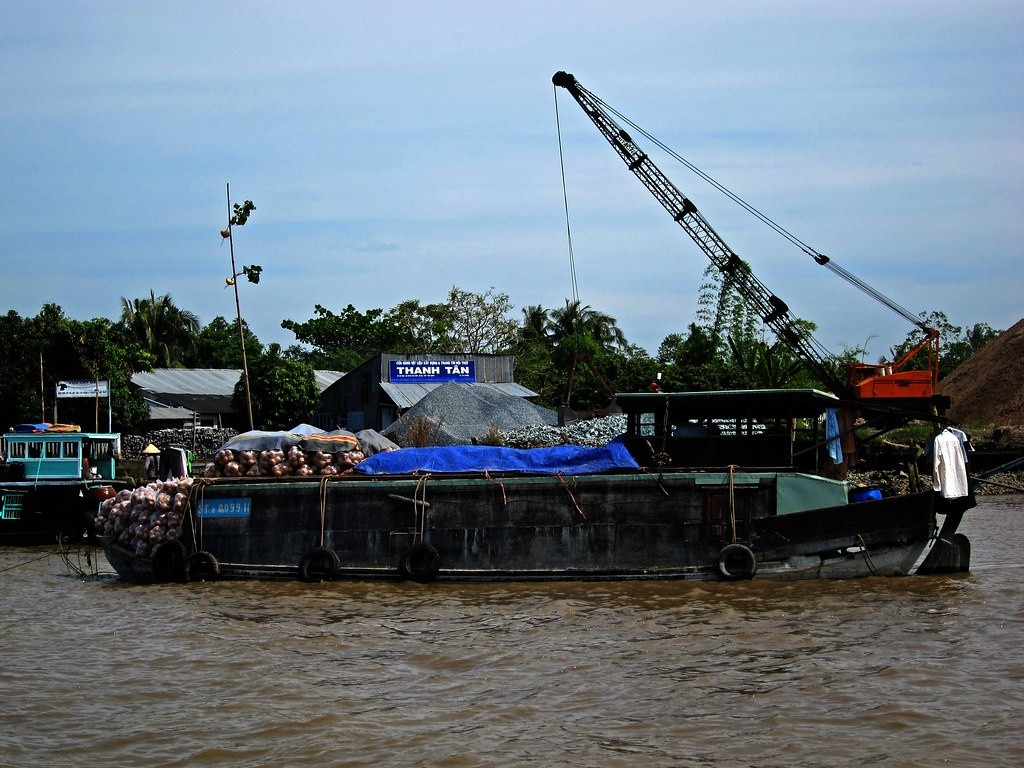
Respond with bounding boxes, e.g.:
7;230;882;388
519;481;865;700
0;423;128;542
92;389;971;582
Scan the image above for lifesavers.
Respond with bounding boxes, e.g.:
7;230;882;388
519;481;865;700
716;543;757;581
401;543;441;583
183;550;221;583
300;545;341;582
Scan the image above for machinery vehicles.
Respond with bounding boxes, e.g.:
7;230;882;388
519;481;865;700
553;70;951;434
